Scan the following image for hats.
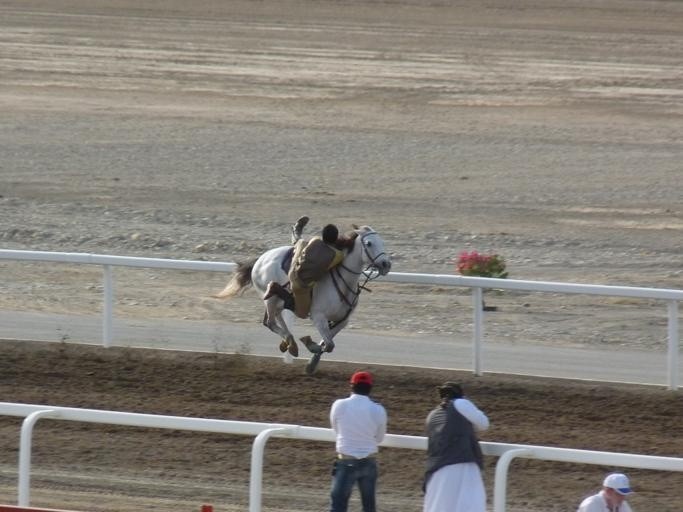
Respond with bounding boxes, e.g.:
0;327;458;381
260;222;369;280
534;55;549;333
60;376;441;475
350;371;372;385
438;381;462;398
603;473;635;495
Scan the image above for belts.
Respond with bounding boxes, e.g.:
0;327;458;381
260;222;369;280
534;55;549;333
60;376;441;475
337;453;355;460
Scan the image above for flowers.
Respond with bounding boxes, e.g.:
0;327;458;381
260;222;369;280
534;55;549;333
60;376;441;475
456;250;505;275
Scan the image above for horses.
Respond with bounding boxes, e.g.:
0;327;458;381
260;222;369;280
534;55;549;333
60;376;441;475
210;223;392;376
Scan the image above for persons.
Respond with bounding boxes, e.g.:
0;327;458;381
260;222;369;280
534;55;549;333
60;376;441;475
330;372;389;512
262;215;344;320
577;472;632;512
422;382;490;512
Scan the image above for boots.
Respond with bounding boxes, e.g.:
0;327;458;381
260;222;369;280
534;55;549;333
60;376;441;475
263;281;290;300
291;216;309;244
